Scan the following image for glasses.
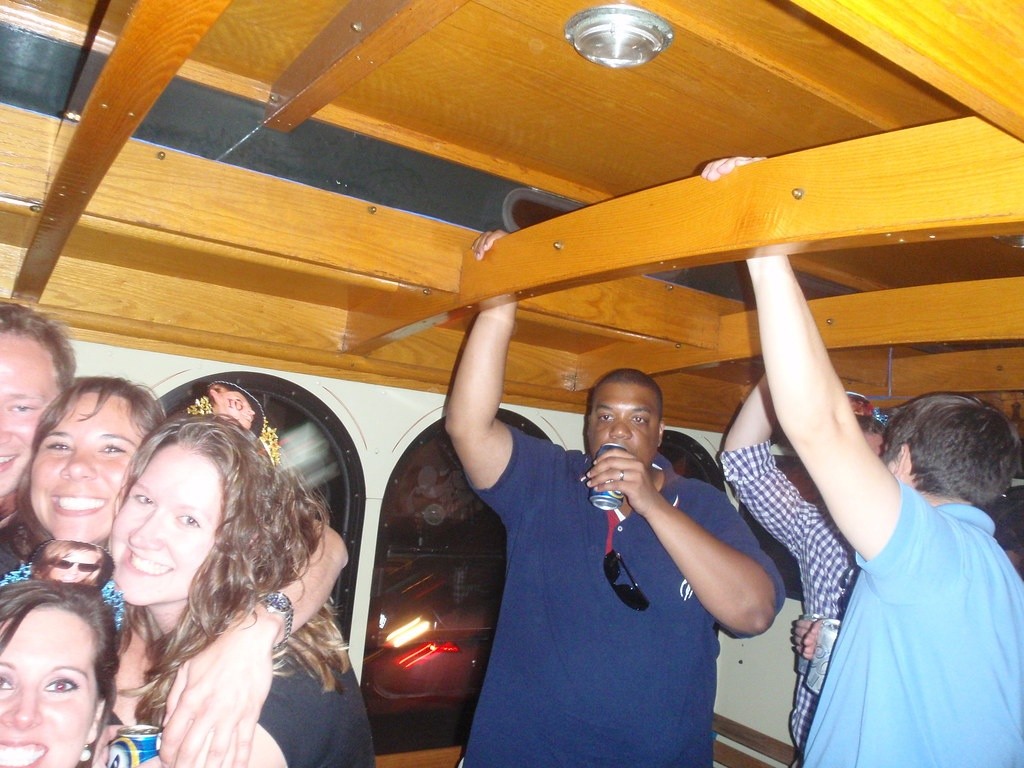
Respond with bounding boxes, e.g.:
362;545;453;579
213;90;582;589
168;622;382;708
50;558;100;572
603;549;649;612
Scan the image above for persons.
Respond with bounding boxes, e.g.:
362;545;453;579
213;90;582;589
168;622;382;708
1;298;379;768
702;156;1023;768
38;540;103;586
211;384;256;431
444;229;789;768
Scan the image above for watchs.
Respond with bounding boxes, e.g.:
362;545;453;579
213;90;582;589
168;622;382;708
260;589;294;653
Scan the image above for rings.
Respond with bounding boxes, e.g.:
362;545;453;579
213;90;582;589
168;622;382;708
619;470;625;481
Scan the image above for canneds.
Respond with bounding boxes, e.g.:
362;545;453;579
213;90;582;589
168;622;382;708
805;619;840;695
106;725;163;768
590;444;630;510
797;613;823;674
